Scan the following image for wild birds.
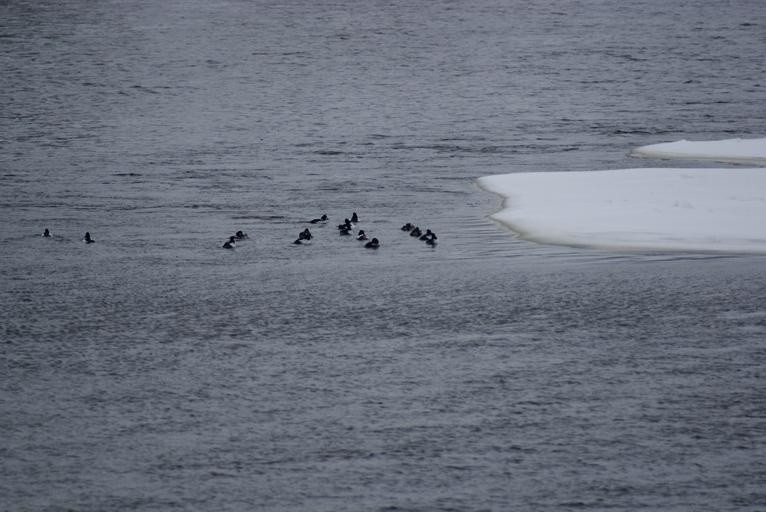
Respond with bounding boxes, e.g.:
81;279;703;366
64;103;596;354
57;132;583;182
337;210;382;250
294;213;329;244
401;222;437;244
40;228;52;237
223;235;238;249
235;230;248;239
82;231;95;244
